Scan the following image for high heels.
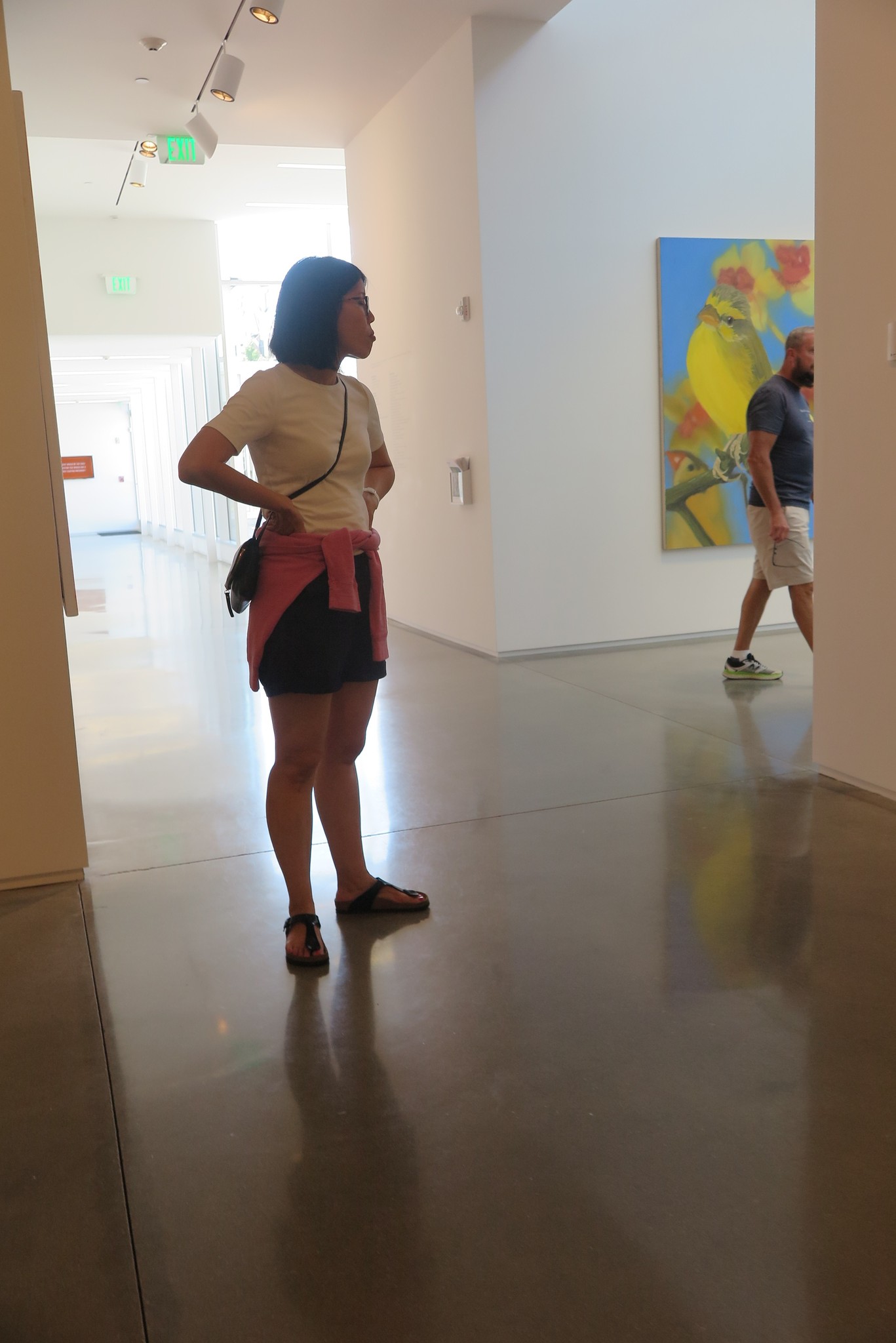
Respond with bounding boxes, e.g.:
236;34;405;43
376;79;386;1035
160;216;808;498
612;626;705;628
334;878;429;912
283;913;329;966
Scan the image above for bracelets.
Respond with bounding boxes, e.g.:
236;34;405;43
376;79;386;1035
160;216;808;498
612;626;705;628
363;487;380;503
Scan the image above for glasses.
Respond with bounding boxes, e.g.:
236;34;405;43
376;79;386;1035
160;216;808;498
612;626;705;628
772;538;805;567
345;296;370;315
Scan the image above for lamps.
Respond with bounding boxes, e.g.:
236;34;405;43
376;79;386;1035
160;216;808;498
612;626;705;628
210;40;245;102
249;0;284;25
184;100;218;159
128;152;147;187
138;136;157;158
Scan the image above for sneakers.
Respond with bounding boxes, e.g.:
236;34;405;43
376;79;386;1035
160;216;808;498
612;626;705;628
722;654;783;680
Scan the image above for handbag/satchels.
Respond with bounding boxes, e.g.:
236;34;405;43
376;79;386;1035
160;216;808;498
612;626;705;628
224;538;258;618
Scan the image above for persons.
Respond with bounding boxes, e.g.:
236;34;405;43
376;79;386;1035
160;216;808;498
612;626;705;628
721;326;814;681
177;254;429;966
